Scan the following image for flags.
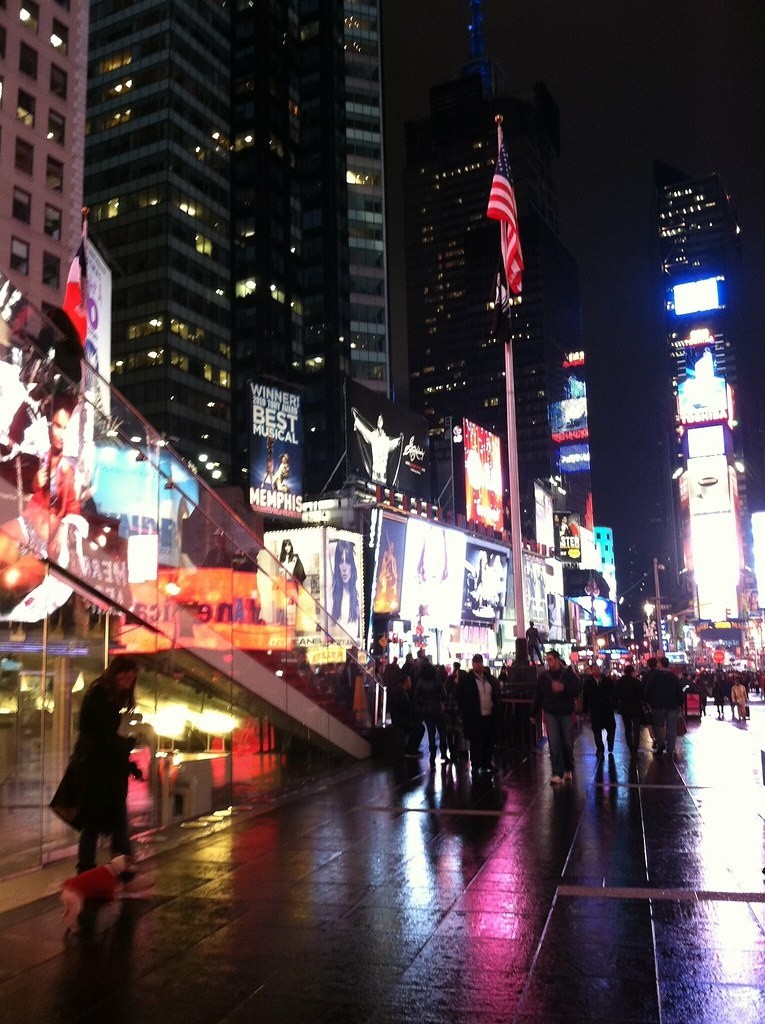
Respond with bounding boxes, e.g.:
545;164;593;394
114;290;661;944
487;133;526;347
62;222;89;351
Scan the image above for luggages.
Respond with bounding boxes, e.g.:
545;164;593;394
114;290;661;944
745;701;749;718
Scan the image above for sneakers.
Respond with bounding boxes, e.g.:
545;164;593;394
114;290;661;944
441;754;451;762
429;751;436;763
122;874;157;893
656;746;666;755
595;744;605;758
652;740;658;748
563;770;573;780
607;741;614;752
549;775;564;784
403;747;424;759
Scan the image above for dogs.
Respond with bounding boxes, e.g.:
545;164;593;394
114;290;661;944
59;854;137;935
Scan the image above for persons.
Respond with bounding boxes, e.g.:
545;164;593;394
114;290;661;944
48;654;155;898
201;528;388;754
2;289;84;473
351;408;403;491
387;616;762;783
559;514;573;548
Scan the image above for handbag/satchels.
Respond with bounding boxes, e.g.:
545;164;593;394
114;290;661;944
676;713;687;736
640;707;654;725
